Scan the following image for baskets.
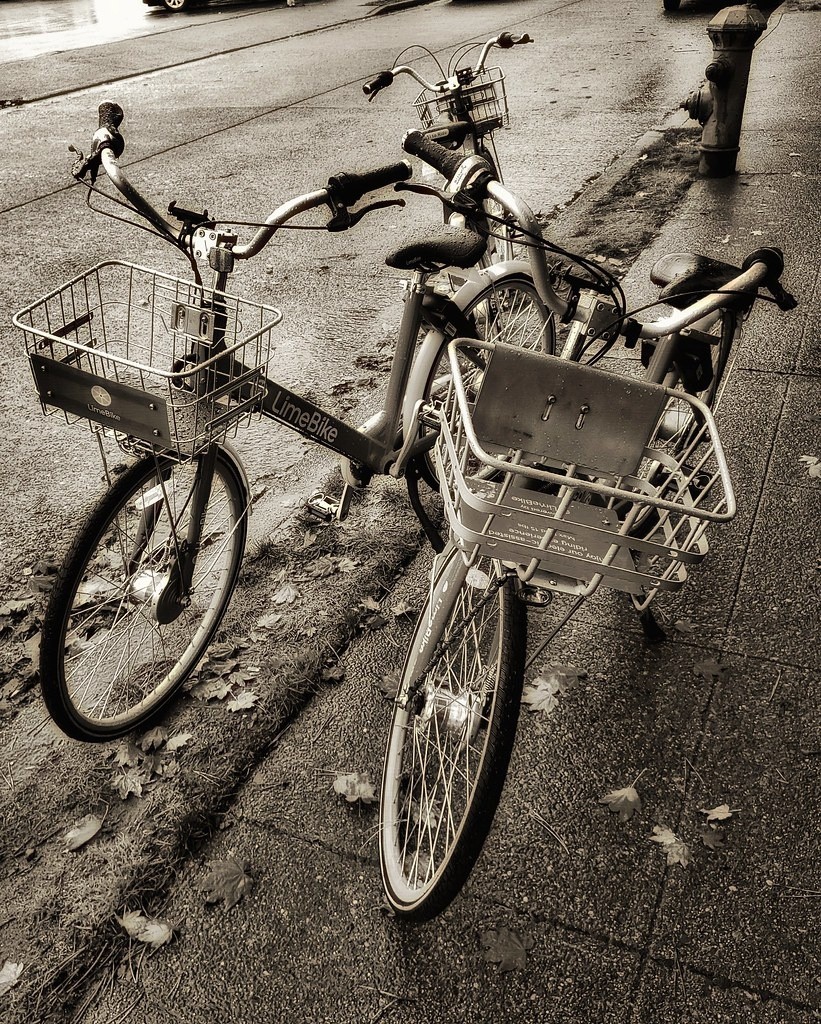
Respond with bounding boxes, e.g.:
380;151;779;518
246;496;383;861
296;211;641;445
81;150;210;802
11;259;283;465
433;337;736;611
411;66;509;134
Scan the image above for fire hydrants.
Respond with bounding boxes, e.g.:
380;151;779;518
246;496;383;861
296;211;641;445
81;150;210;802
680;5;768;181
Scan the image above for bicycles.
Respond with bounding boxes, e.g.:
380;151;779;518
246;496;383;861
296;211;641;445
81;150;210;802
12;102;557;743
362;30;534;324
377;129;800;925
